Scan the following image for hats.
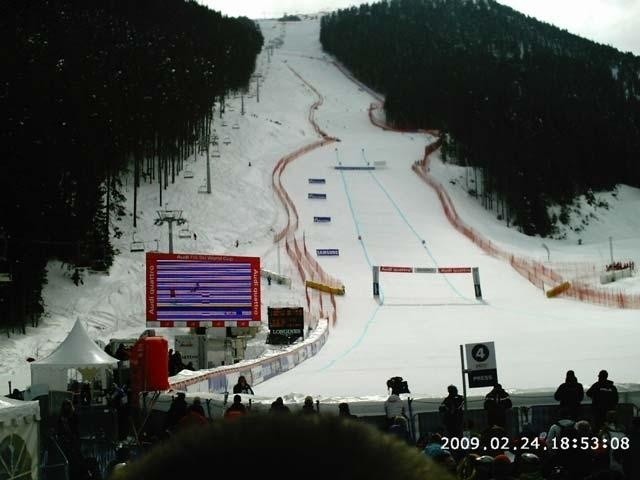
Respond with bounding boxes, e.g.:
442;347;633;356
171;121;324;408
475;452;539;470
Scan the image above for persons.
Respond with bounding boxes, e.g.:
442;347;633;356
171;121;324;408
178;406;208;430
167;349;195;377
224;395;246;421
298;398;320;415
103;343;133;360
336;403;358;420
188;398;206;417
167;392;187;426
271;397;292;416
57;400;85;479
106;383;129;441
384;371;640;480
233;376;254;394
